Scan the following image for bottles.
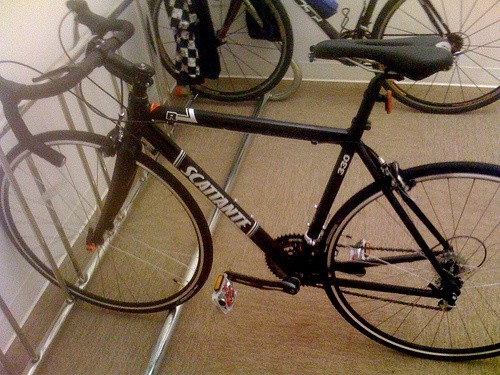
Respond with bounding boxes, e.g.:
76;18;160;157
304;0;339;19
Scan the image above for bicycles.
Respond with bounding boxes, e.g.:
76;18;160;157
148;1;500;116
0;0;499;362
